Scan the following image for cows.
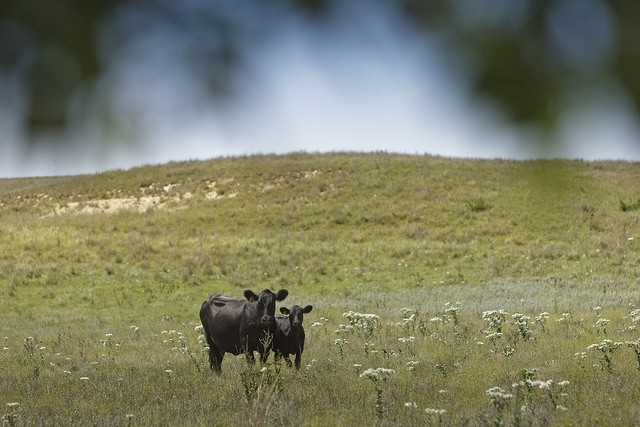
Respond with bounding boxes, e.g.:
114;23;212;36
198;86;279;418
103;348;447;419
271;304;313;375
199;288;289;375
